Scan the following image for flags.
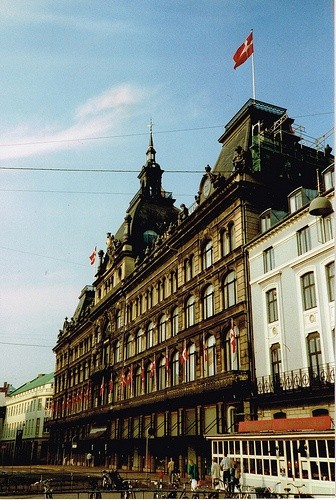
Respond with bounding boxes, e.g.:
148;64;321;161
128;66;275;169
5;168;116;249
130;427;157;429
140;361;145;381
165;349;170;372
52;366;133;412
229;320;236;360
149;356;156;378
233;32;254;70
89;250;96;265
181;343;188;363
202;334;208;361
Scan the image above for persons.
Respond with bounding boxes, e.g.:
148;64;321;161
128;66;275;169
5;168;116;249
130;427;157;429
167;458;180;481
178;203;189;222
210;453;241;492
232;144;249;171
188;459;199;490
86;452;92;466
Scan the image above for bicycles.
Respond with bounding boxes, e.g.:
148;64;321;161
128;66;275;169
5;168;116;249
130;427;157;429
150;479;306;499
87;475;102;499
32;478;56;499
120;479;139;499
